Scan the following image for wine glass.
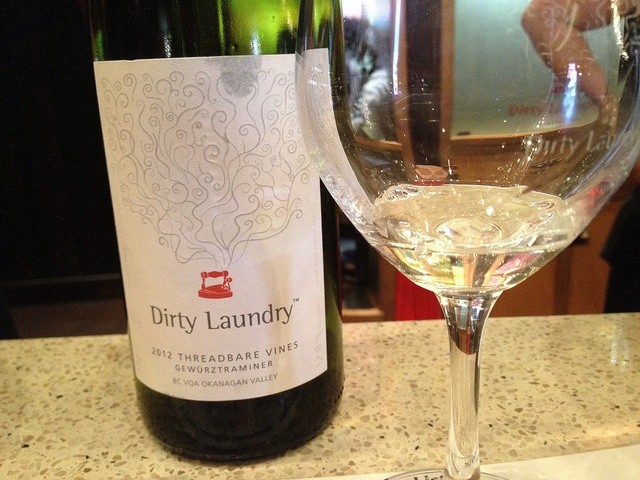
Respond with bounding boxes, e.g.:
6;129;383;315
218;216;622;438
296;1;640;480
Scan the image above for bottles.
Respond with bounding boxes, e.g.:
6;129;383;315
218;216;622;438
79;1;345;464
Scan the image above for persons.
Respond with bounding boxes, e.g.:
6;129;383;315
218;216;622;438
520;0;639;312
